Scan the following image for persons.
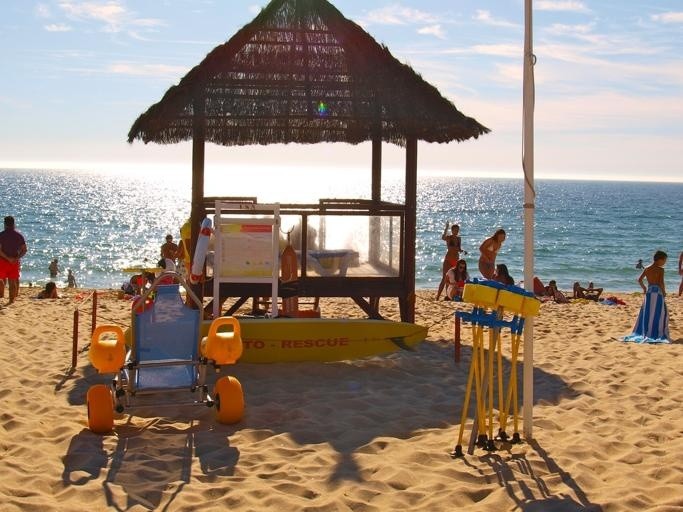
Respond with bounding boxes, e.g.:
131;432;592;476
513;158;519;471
492;264;515;286
0;216;27;303
478;228;506;279
37;282;60;299
121;271;156;295
569;281;603;300
177;229;184;264
63;270;78;288
678;251;683;296
160;234;179;265
637;250;668;336
636;259;644;269
157;258;178;286
544;279;569;304
443;259;470;301
49;258;61;277
435;219;468;302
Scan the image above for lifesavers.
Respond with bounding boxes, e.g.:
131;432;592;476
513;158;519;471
190;218;212;285
280;247;299;283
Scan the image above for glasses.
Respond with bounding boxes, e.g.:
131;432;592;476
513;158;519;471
459;266;465;269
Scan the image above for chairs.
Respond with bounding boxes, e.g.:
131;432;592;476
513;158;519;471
110;271;214;413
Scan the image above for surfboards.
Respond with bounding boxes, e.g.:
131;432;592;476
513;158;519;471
125;318;429;365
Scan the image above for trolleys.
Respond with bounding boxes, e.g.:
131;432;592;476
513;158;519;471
86;270;244;433
